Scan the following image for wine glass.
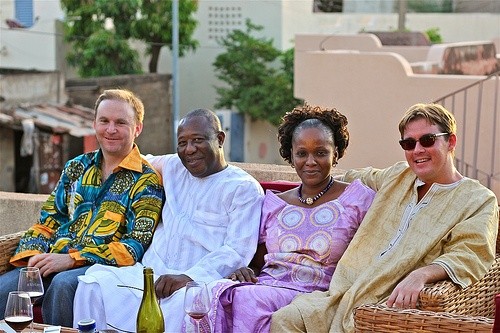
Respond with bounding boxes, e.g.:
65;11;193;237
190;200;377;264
18;267;45;333
183;281;211;333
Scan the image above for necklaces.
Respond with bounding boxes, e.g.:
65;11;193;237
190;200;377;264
297;176;334;205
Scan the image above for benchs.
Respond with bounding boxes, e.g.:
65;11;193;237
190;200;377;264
0;182;500;317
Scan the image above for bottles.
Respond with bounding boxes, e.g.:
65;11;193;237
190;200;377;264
135;268;166;333
77;318;97;333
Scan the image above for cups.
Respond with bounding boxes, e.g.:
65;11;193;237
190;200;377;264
4;290;33;333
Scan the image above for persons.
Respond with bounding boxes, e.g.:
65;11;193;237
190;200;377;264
181;105;376;333
270;103;499;333
0;90;164;328
73;108;264;333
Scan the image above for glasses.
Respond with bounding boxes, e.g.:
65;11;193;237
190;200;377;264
399;132;447;150
